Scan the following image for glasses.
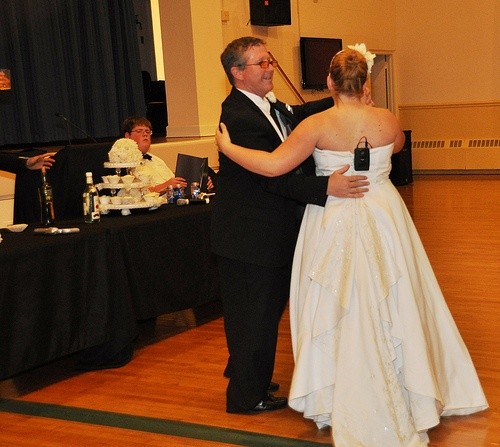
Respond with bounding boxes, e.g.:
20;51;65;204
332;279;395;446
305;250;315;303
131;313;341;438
231;60;278;69
128;129;153;136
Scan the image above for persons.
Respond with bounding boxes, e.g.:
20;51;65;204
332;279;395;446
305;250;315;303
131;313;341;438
121;116;214;198
215;43;489;447
210;37;369;414
0;152;57;174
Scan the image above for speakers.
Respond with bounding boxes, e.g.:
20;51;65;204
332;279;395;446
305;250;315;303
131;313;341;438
249;0;291;26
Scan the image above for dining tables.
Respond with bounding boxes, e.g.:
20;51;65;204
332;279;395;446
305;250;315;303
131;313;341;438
0;173;222;396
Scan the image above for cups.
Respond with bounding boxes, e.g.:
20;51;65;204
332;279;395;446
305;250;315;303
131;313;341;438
191;182;200;199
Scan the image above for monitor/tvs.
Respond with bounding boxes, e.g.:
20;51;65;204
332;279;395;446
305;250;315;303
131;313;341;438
300;36;343;90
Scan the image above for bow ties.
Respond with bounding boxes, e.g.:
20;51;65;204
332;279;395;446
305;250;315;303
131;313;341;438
142;154;153;161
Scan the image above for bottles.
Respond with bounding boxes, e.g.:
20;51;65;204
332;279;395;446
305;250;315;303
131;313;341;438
167;183;183;204
82;171;100;224
38;167;55;226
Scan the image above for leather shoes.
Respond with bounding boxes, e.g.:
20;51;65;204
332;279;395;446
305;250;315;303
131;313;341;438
223;366;280;392
225;392;289;414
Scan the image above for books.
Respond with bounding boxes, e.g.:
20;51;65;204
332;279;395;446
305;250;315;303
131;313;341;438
175;153;209;194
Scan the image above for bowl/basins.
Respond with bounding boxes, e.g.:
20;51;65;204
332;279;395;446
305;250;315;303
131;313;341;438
99;174;160;205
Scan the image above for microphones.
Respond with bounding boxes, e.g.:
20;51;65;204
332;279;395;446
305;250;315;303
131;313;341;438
54;112;97;144
